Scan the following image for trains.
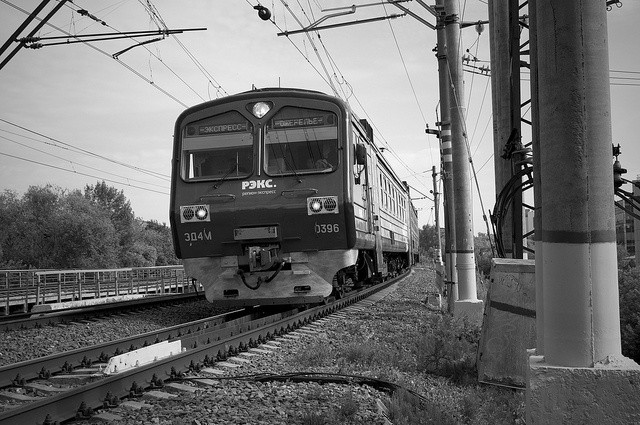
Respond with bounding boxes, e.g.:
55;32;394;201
169;87;419;305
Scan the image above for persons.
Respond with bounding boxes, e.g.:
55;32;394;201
306;143;333;168
